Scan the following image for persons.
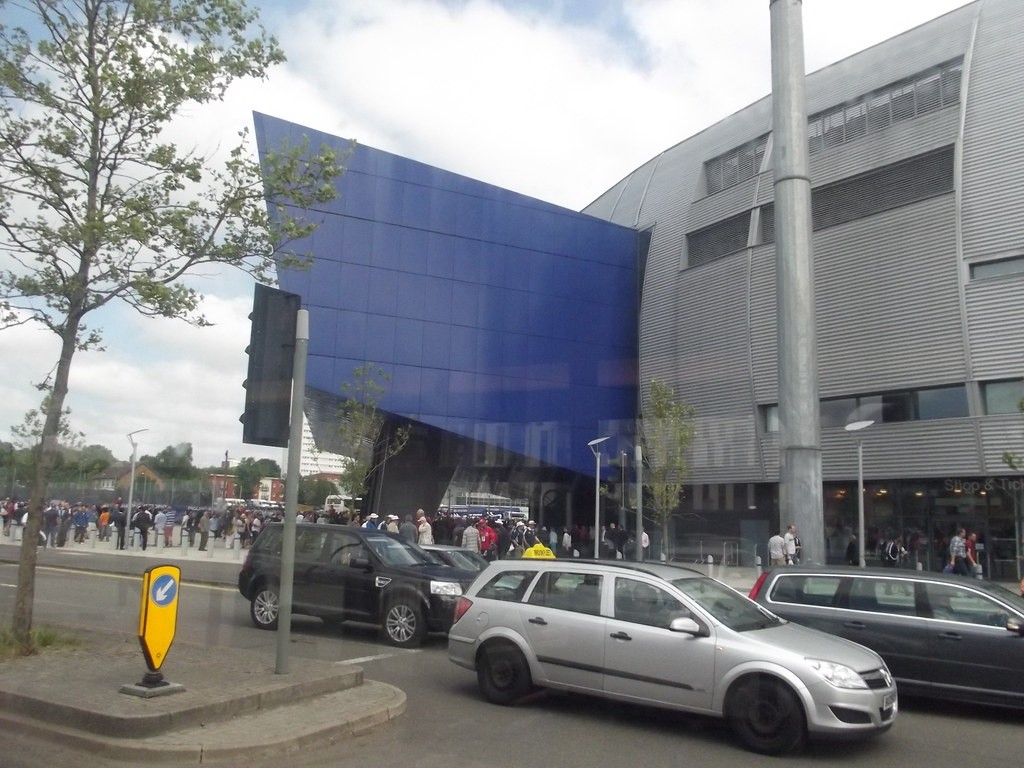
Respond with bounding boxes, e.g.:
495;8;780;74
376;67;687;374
846;532;860;566
767;524;802;566
948;527;978;579
882;533;904;568
0;493;650;562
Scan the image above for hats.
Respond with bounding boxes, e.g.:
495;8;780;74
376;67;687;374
528;520;536;524
391;516;399;521
417;517;426;522
479;519;485;524
387;514;394;517
370;513;378;518
515;521;525;528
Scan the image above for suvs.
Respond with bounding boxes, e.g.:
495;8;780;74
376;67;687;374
239;523;498;650
743;564;1024;710
447;543;900;758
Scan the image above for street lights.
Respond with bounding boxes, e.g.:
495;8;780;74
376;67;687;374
588;437;613;558
845;420;877;566
122;427;151;551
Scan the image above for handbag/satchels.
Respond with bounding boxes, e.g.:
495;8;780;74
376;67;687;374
0;507;8;516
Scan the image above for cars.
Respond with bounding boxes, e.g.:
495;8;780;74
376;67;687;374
362;543;526;591
